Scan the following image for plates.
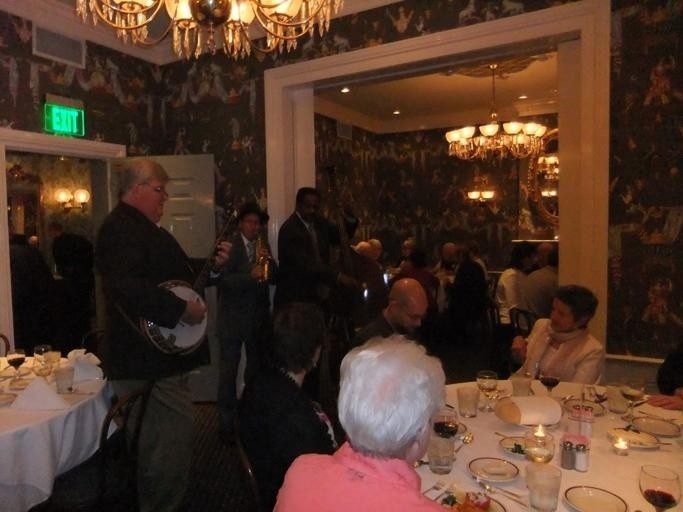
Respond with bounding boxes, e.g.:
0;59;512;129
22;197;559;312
0;365;35;405
429;399;682;511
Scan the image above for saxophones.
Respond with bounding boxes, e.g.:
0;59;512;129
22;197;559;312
255;232;272;285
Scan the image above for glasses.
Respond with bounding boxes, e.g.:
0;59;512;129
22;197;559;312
391;298;428;321
137;181;163;194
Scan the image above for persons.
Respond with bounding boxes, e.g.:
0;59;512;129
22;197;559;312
95;153;234;507
214;186;683;512
7;216;94;357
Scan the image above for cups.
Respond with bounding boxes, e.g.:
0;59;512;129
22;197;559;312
457;386;478;418
54;367;74;395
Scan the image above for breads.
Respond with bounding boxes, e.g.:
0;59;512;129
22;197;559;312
494;396;521;426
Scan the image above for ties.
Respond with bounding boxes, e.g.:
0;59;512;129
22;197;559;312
247;240;256;265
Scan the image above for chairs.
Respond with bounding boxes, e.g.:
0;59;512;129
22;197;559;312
451;272;502;323
509;308;537;332
93;380;153;512
232;414;259;495
0;334;10;357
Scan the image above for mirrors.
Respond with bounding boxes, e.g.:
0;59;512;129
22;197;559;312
7;164;43;248
515;112;559;240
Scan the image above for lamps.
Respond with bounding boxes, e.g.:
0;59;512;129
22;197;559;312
55;188;90;214
445;64;546;161
75;0;344;62
469;176;495;206
538;154;559;199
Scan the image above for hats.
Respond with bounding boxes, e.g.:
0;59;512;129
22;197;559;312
237;203;269;226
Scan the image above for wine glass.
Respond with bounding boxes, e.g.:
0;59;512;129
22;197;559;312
7;344;52;390
475;366;647;420
